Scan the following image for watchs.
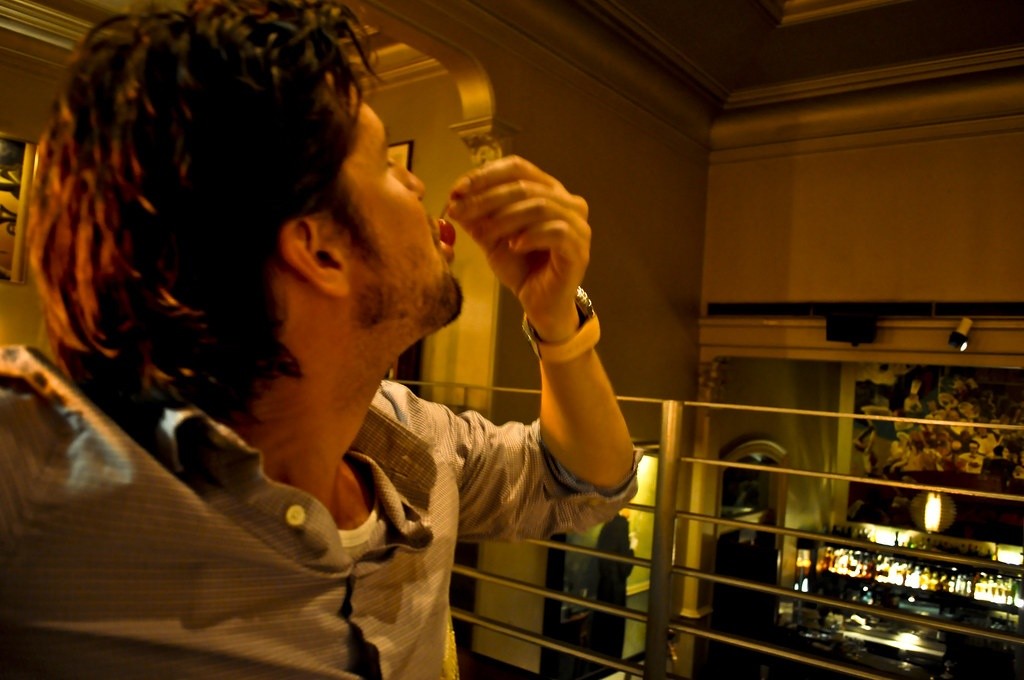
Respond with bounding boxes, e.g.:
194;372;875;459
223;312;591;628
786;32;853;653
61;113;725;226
520;285;601;363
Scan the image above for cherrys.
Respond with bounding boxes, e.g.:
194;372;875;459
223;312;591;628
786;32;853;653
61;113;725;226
438;219;455;246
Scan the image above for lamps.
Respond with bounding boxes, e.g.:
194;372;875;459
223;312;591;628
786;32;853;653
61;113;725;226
948;316;974;348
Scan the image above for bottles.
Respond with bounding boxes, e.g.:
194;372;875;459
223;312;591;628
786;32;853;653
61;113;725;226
819;522;1024;609
967;637;1017;671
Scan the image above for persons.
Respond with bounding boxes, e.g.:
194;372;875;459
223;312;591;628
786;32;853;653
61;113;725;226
0;1;642;680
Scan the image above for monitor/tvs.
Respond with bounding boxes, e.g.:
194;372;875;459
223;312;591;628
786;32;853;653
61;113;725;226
826;303;879;343
